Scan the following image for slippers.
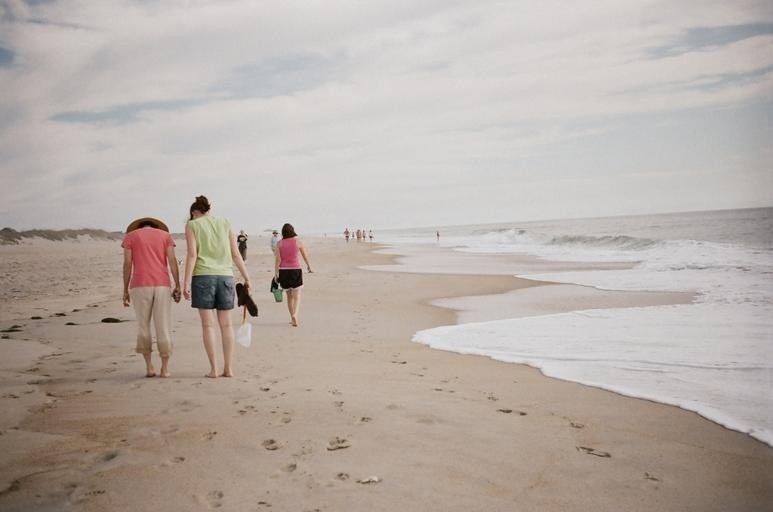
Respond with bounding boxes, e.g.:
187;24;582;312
235;282;259;318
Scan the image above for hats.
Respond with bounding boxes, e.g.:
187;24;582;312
125;215;170;234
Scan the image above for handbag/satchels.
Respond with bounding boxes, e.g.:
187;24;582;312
237;302;252;348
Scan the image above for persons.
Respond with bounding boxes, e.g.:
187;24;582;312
237;230;248;261
271;230;282;256
274;223;313;326
343;227;374;242
121;218;181;378
183;195;250;377
